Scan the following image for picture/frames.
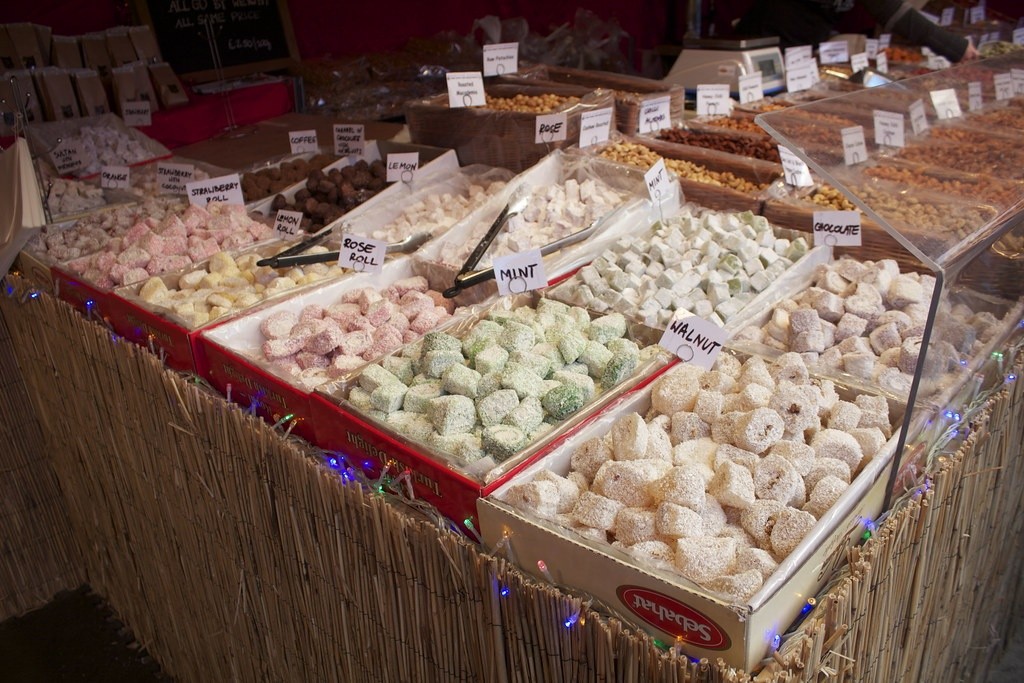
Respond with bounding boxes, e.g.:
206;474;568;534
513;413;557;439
129;0;302;85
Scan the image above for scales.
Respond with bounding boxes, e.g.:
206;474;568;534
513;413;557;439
659;31;789;100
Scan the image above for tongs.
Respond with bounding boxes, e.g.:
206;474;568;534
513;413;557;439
442;181;604;299
256;220;432;269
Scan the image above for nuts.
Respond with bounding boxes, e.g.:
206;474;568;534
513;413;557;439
472;39;1023;264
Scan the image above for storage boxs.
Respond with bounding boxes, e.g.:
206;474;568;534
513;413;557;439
1;22;1024;681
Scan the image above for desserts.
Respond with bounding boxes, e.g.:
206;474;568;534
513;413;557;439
25;120;1003;609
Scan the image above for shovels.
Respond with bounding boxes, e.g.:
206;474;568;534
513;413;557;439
819;68;910;95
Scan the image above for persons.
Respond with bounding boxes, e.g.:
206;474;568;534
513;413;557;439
735;0;981;65
